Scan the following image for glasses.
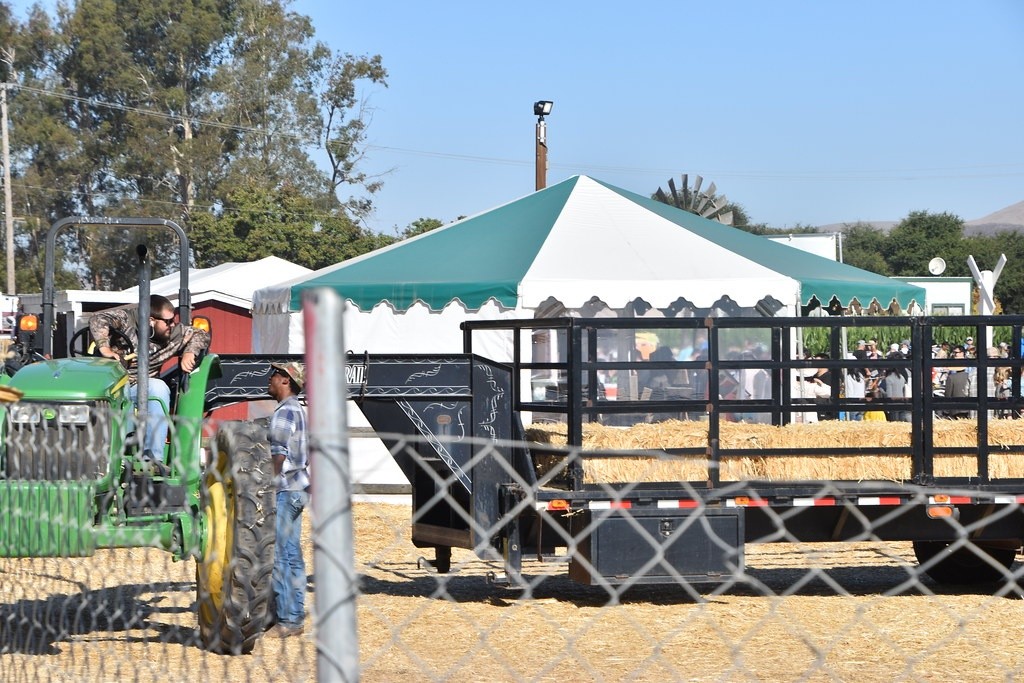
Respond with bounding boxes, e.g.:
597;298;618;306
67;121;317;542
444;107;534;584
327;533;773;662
270;369;288;378
150;316;174;325
953;351;962;355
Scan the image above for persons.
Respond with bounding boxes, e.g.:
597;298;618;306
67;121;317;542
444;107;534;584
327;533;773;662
265;362;312;638
87;294;211;461
597;336;1024;423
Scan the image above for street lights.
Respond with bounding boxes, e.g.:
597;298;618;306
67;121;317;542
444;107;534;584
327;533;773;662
532;99;555;380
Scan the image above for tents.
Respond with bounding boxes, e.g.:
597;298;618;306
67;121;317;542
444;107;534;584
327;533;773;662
250;176;925;505
112;257;313;443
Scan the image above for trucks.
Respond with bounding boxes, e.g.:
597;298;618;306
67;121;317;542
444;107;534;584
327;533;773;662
350;308;1024;588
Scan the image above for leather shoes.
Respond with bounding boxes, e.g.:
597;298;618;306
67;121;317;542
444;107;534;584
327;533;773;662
265;623;304;638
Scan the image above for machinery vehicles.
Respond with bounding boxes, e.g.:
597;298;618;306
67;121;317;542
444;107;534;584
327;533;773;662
0;213;280;658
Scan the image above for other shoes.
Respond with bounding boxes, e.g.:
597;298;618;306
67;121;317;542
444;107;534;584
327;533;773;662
154;463;177;476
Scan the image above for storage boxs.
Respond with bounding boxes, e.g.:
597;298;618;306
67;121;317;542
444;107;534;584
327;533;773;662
569;508;746;584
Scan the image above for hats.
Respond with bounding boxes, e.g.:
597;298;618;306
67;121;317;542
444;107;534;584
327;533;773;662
272;362;306;387
967;336;973;341
900;340;910;346
888;343;900;350
796;368;819;377
856;340;865;345
987;347;1002;357
865;340;876;345
967;345;975;350
